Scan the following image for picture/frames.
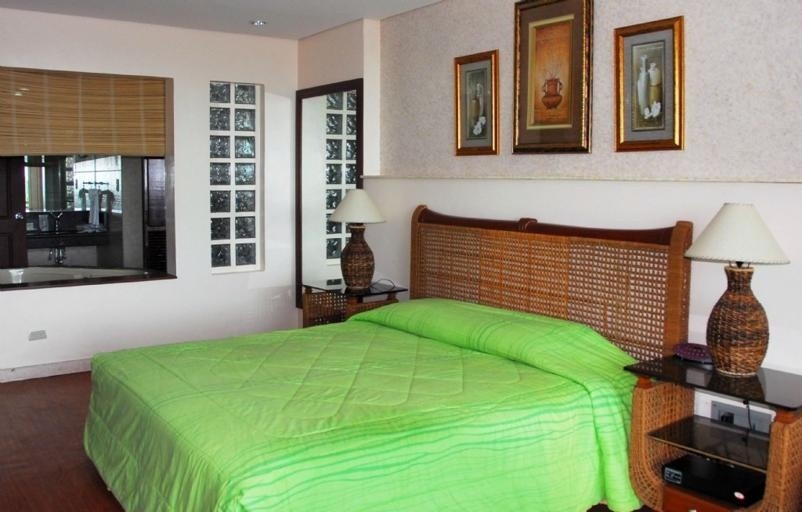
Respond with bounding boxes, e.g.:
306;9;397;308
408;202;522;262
613;15;685;153
512;0;595;155
453;49;502;157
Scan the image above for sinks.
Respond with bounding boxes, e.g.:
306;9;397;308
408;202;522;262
27;230;73;236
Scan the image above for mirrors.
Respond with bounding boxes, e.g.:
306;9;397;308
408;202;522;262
24;155;96;215
294;76;364;311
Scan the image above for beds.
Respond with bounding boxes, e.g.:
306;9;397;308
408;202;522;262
85;203;693;511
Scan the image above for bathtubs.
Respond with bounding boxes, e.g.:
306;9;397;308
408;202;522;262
0;266;149;286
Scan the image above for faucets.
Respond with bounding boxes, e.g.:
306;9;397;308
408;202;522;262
49;207;65;232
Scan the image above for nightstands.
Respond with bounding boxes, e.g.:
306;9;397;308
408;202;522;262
296;278;408;328
622;356;802;512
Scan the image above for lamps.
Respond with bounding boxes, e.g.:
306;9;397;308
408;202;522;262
681;202;791;378
328;189;385;289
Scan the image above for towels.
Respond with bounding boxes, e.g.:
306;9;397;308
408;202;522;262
81;193;86;211
88;190;100;225
